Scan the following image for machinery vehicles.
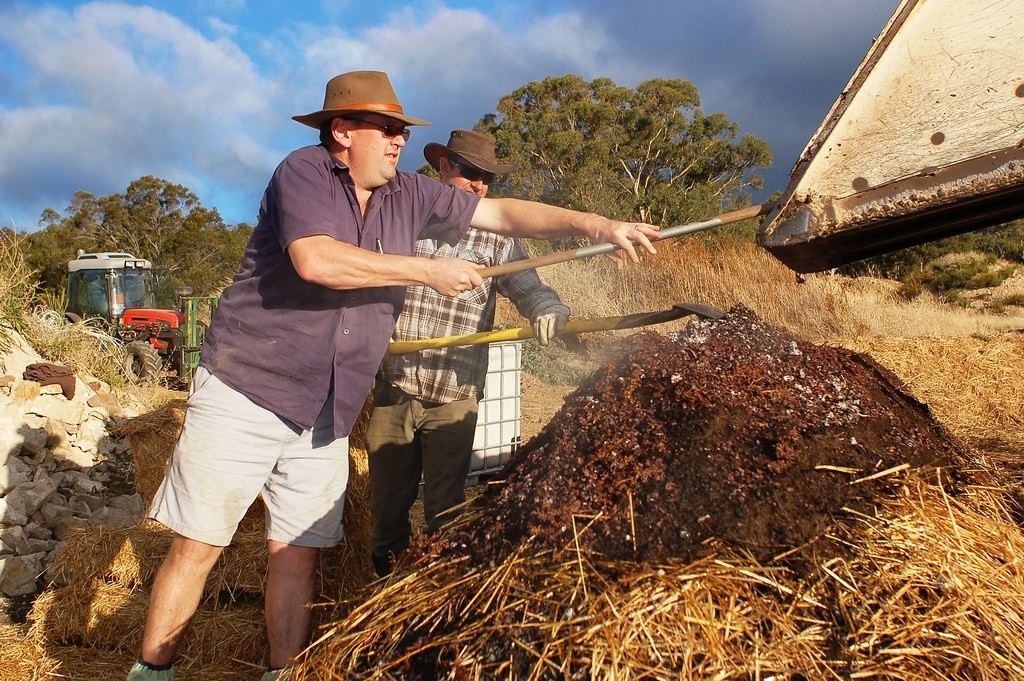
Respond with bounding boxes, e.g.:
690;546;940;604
63;247;209;389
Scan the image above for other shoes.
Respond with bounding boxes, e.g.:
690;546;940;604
124;658;175;681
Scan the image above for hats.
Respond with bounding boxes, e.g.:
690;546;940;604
422;129;516;174
292;71;432;131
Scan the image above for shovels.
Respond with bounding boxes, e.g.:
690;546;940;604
479;201;774;285
386;300;729;354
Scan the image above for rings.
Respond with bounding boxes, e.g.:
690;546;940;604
634;225;640;230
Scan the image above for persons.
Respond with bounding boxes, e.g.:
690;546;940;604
373;128;573;575
127;68;666;681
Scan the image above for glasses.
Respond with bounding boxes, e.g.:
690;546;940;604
346;116;411;143
447;159;497;186
